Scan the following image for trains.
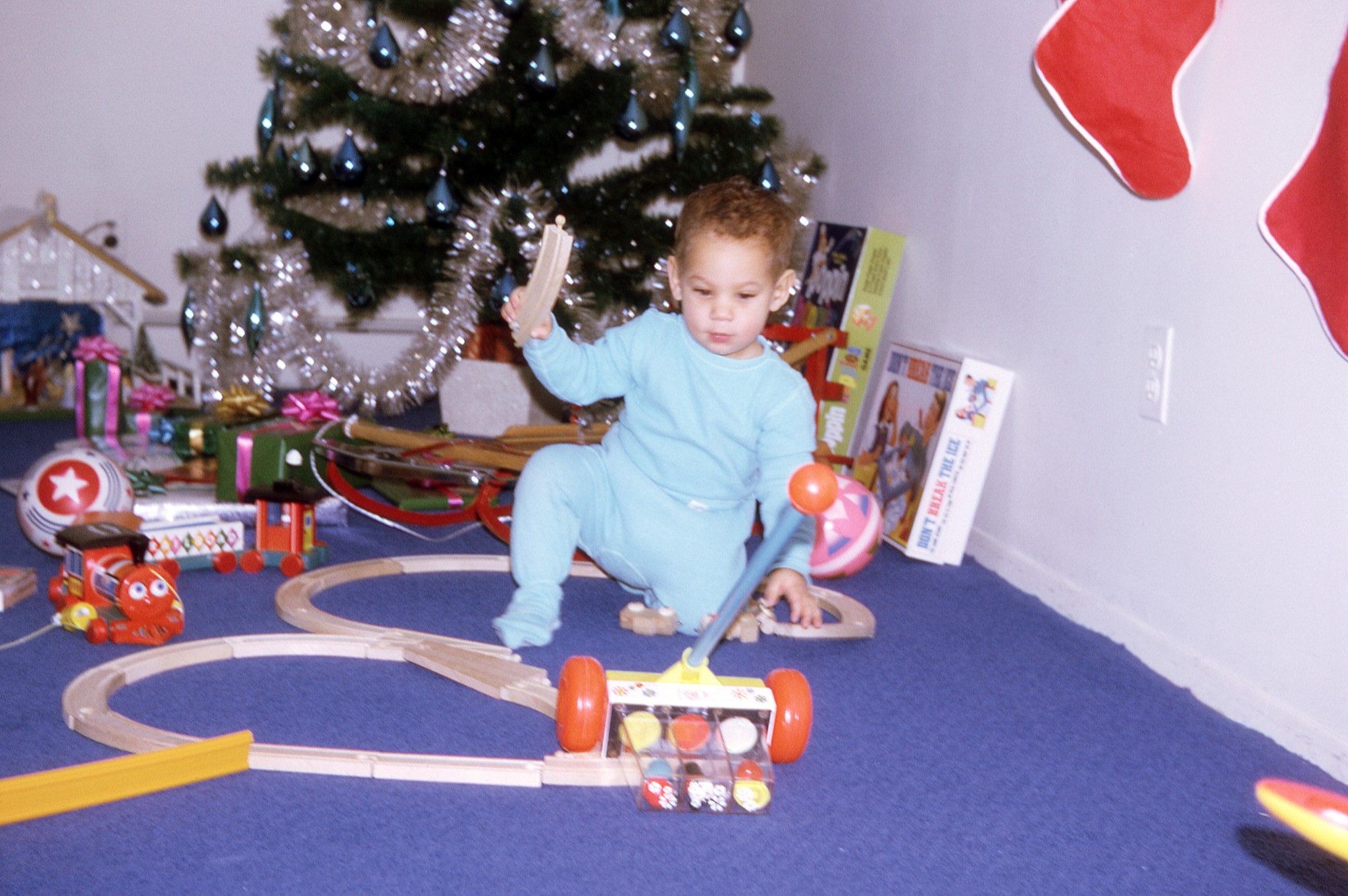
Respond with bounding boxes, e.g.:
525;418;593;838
49;478;335;642
